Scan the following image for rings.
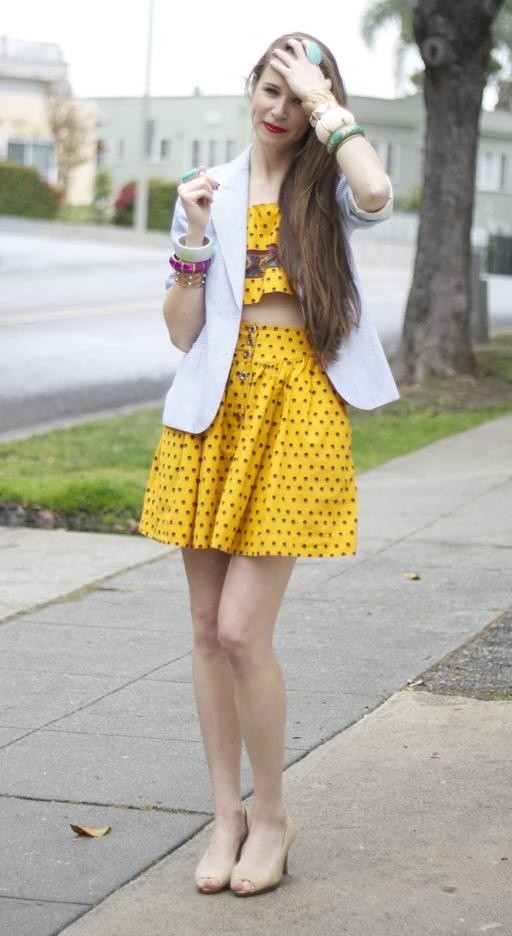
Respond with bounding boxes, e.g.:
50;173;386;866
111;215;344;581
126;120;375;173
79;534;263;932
179;168;202;183
307;41;322;66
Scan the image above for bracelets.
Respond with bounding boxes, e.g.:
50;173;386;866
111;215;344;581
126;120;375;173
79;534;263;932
174;271;206;287
315;108;354;141
301;90;339;112
324;125;365;154
168;256;211;274
172;232;215;264
307;102;339;125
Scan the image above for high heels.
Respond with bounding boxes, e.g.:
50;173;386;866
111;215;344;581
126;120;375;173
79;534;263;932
191;801;248;898
226;805;296;900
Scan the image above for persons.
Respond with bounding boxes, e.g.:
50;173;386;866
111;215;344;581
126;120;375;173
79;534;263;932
136;29;401;898
